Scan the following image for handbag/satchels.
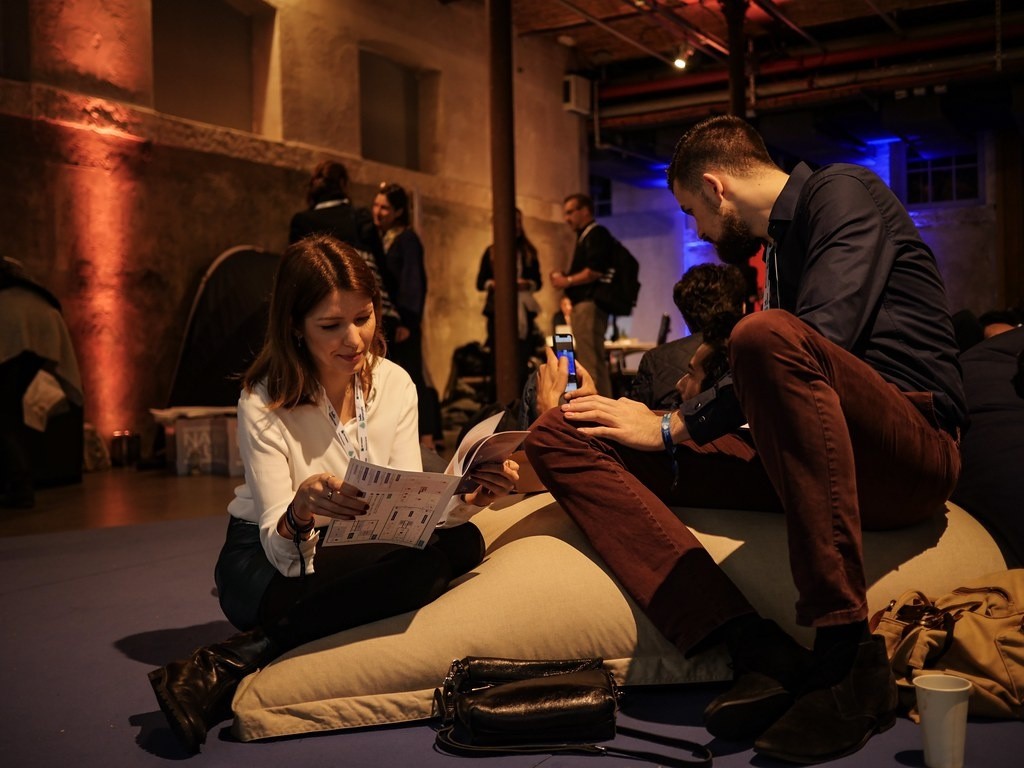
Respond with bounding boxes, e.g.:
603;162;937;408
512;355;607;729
430;655;713;768
867;565;1024;721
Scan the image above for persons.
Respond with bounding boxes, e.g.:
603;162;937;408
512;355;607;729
636;262;743;412
147;232;521;751
950;310;984;354
524;112;967;765
534;310;787;516
549;192;613;399
288;159;384;281
372;184;447;456
477;206;542;356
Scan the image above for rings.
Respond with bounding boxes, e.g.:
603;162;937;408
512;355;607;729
328;490;333;501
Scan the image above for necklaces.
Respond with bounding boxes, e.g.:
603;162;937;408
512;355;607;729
384;234;393;246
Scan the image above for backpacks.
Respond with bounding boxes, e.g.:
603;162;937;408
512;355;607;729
577;223;642;317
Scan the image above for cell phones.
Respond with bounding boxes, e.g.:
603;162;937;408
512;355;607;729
554;333;577;395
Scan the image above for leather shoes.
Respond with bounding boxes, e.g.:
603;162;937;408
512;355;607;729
752;631;899;764
704;619;835;744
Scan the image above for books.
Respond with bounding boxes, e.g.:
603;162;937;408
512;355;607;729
322;410;532;549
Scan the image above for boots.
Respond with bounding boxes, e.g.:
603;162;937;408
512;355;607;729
148;628;277;757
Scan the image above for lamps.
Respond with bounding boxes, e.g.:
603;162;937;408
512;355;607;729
675;45;695;68
110;431;140;466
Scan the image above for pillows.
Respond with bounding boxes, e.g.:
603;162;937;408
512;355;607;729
229;490;1009;741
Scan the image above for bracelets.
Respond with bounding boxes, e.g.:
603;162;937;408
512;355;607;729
283;500;315;581
662;412;674;449
567;276;573;284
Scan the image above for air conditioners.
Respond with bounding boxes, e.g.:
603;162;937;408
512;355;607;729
564;76;591;114
